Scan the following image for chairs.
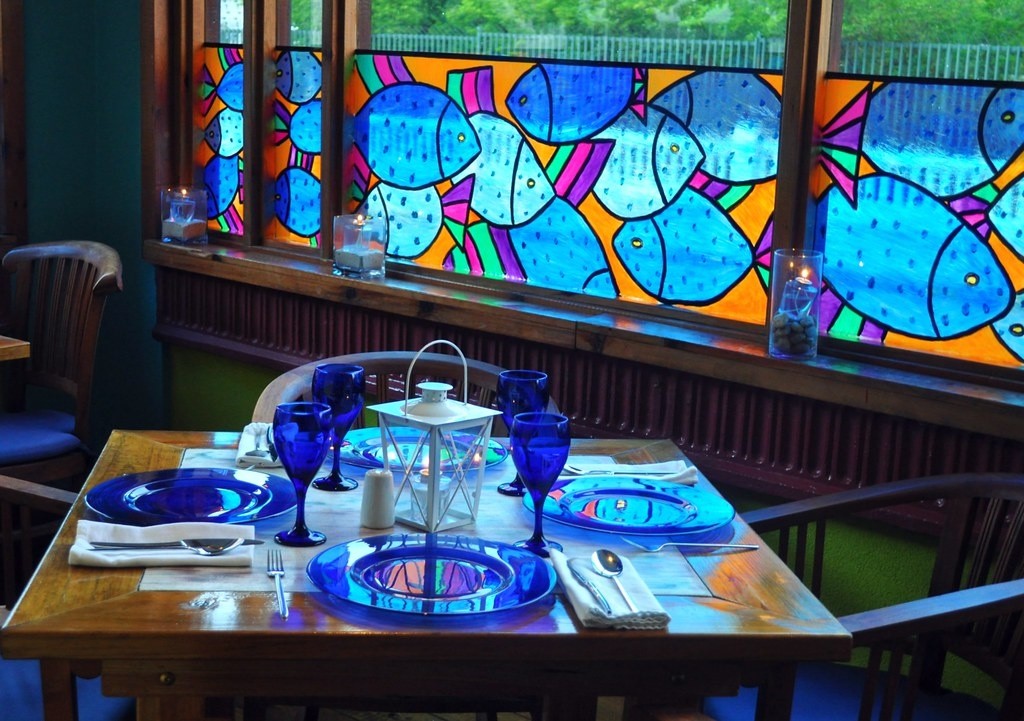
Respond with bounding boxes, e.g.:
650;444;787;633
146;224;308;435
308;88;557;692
240;350;565;719
1;239;123;612
731;471;1024;721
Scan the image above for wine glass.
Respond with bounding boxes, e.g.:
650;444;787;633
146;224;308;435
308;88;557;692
506;410;574;557
495;370;552;495
311;362;367;492
272;402;336;548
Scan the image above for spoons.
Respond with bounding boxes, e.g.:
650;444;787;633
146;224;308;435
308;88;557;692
592;548;640;614
86;538;244;557
245;427;270;458
562;464;678;476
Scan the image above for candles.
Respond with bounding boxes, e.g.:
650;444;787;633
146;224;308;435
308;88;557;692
342;215;370;250
778;267;818;322
170;188;196;225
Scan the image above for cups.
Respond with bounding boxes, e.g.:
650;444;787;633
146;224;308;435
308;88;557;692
331;214;386;281
161;187;210;247
769;247;824;362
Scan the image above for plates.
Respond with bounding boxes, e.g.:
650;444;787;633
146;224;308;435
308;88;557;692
521;476;736;533
306;532;557;614
327;425;508;472
82;468;300;525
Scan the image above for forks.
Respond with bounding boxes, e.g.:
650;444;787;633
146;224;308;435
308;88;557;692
265;549;292;619
618;535;762;552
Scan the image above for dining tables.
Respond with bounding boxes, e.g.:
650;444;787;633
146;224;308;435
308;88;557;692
0;427;852;721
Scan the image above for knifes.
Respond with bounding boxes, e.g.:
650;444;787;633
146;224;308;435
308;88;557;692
566;558;611;613
265;425;278;462
88;536;264;550
